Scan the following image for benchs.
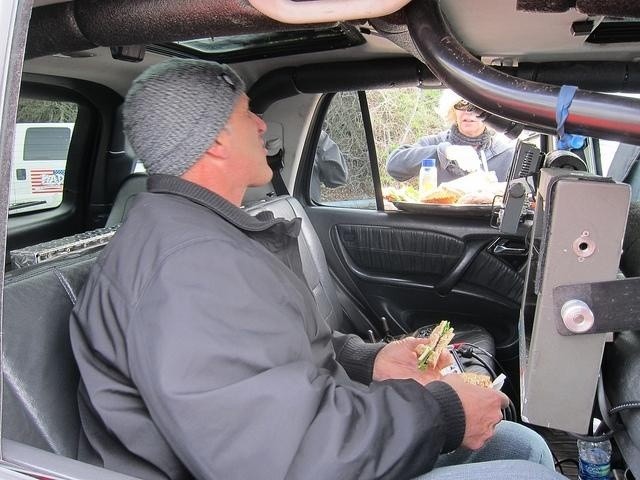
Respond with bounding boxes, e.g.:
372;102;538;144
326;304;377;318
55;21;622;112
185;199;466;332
7;198;497;480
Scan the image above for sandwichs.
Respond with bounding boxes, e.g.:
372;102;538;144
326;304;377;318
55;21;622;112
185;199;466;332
415;319;455;369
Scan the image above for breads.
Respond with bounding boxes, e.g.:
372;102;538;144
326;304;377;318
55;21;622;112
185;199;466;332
459;373;490;389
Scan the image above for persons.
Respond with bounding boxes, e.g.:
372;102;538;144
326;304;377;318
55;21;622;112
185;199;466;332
386;88;515;188
69;58;571;480
309;131;348;202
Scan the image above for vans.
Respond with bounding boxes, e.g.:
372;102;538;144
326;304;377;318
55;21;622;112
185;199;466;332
9;121;80;213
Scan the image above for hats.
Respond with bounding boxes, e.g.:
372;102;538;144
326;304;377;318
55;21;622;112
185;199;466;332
120;56;246;180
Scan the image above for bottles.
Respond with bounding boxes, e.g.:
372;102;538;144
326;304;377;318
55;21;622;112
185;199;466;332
577;417;612;480
419;159;438;199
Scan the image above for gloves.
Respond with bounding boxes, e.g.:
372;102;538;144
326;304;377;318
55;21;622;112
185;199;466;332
445;144;484;171
